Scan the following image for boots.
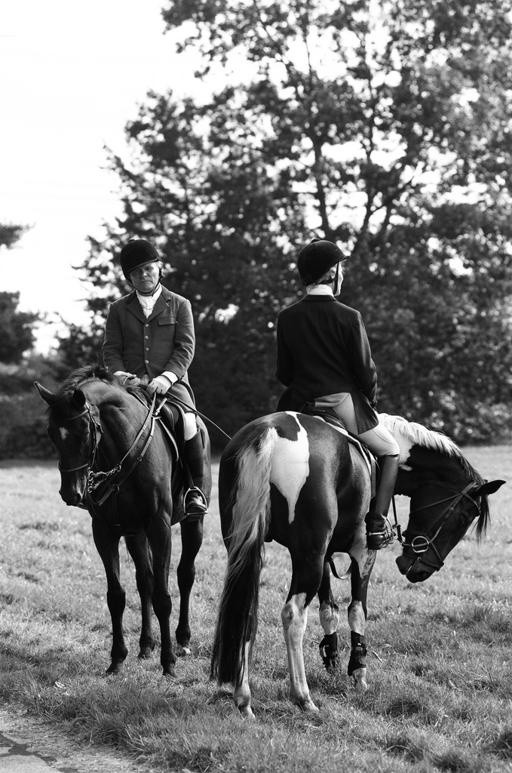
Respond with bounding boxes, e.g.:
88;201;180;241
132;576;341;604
367;455;400;550
181;429;207;513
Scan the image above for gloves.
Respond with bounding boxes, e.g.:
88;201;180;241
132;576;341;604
113;370;140;388
146;370;178;394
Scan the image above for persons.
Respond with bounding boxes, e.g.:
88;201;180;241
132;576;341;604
101;238;210;517
273;238;402;551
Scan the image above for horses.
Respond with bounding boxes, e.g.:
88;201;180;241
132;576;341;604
208;409;507;721
34;363;213;679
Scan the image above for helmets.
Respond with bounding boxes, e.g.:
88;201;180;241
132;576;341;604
299;241;345;285
121;242;160;277
